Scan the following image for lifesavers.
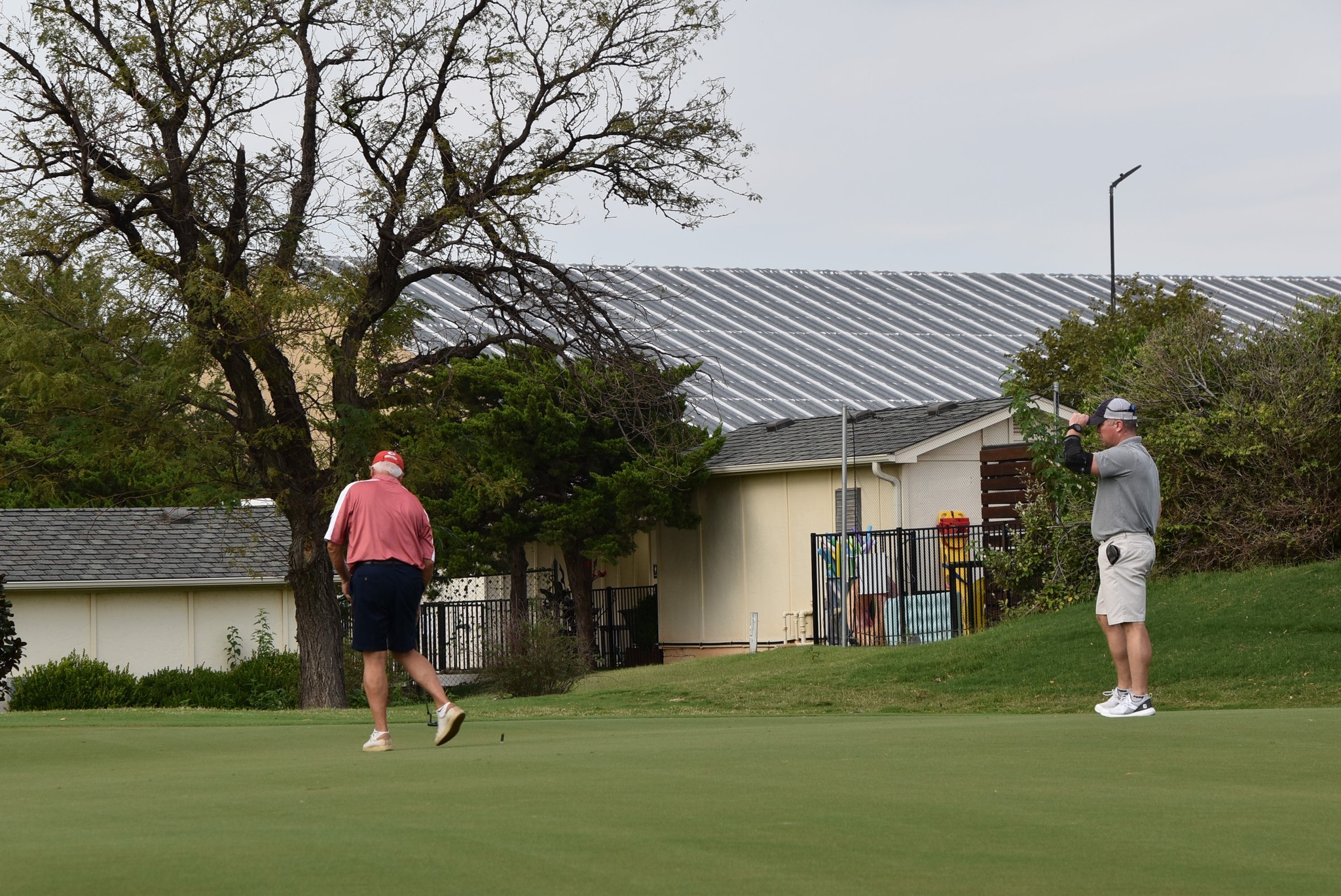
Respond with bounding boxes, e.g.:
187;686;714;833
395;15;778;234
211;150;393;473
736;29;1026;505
846;576;898;646
816;525;876;618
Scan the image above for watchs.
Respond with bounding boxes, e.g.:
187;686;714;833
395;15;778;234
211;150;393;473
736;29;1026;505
1068;424;1082;434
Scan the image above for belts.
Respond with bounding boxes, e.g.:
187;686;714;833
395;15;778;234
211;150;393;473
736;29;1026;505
359;559;407;565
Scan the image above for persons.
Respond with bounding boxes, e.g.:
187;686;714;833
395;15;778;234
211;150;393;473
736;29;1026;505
1062;399;1162;720
325;450;466;751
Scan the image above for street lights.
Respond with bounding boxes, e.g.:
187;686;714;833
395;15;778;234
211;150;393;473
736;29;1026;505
1109;165;1143;310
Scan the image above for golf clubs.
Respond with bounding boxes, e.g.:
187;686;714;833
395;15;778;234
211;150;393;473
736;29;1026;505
414;638;438;727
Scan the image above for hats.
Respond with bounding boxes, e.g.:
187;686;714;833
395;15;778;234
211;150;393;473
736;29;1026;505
371;450;404;472
1087;397;1137;426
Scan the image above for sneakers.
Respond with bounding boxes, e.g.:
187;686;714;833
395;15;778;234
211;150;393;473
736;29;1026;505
1094;688;1126;714
435;702;465;746
362;729;393;751
1100;693;1155;718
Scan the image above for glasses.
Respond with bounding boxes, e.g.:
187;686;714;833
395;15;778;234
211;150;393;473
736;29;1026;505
1106;403;1135;412
